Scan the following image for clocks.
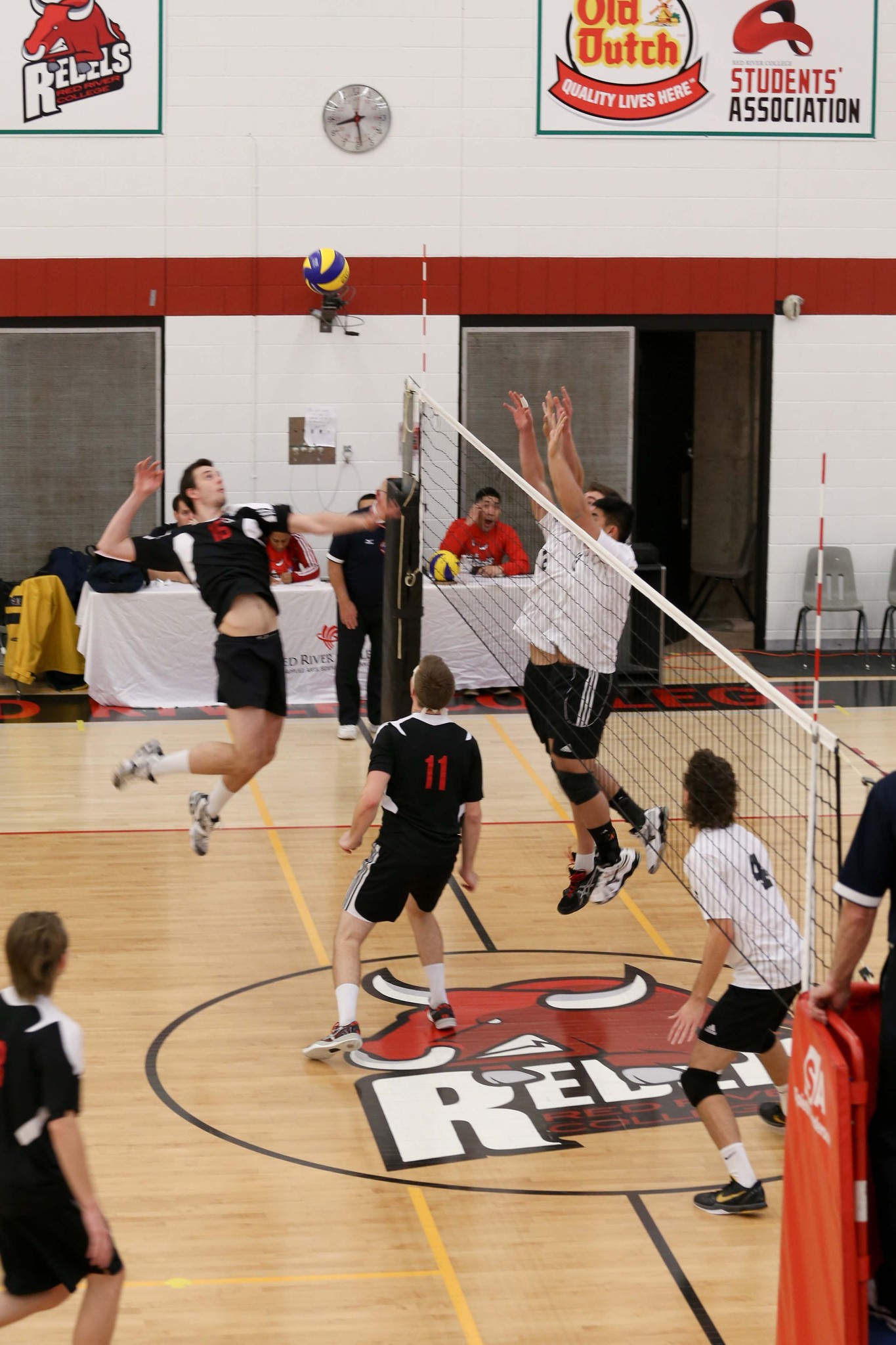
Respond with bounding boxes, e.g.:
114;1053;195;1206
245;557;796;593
323;85;391;152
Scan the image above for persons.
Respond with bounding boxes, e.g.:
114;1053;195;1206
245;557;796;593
303;654;483;1060
502;385;667;915
805;770;896;1345
0;912;128;1344
438;486;531;578
265;531;320;586
146;494;194;584
96;454;401;856
326;494;386;740
667;747;803;1215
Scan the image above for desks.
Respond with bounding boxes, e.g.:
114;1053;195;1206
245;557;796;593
73;575;540;708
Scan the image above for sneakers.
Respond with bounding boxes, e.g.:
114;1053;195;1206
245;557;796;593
190;790;222;857
758;1101;787;1129
369;724;381;733
302;1021;364;1060
588;844;642;905
557;844;604;915
692;1175;768;1215
629;806;669;874
112;738;165;790
426;1003;458;1030
338;724;359;740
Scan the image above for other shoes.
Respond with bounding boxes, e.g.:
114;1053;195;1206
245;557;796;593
493;686;511;695
866;1280;896;1332
462;689;480;696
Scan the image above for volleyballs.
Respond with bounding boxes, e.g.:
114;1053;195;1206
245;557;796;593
429;550;460;582
302;248;350;294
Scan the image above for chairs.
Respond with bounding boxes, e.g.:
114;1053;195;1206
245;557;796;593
877;549;896;668
681;525;754;630
792;546;872;670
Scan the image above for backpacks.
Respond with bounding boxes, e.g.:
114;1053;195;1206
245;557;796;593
33;546;90;611
85;545;151;595
